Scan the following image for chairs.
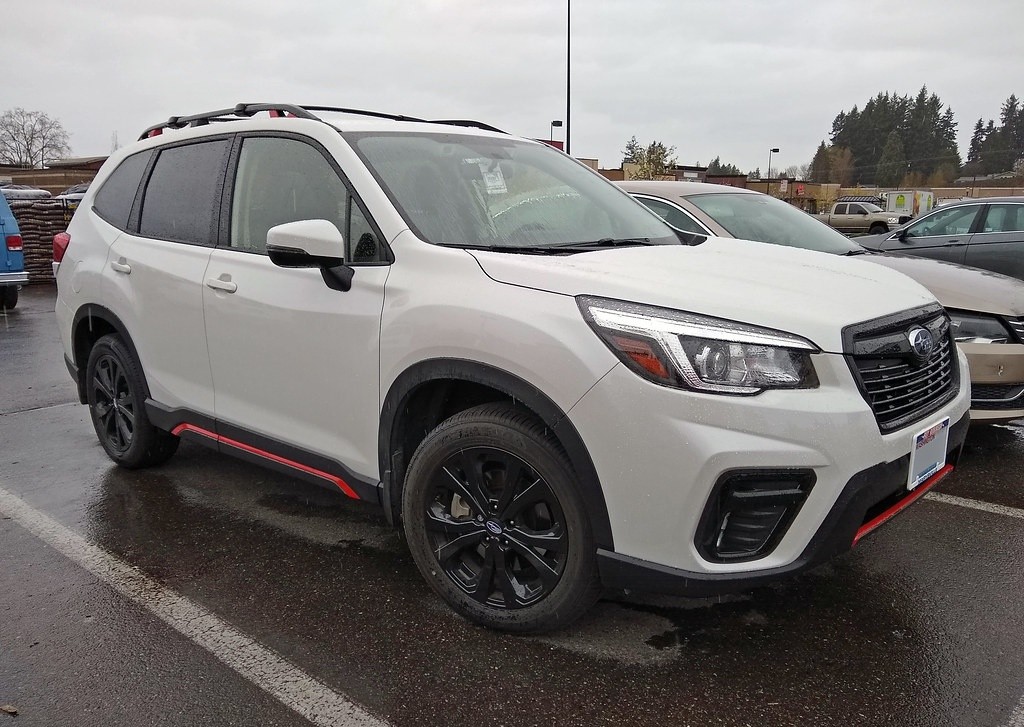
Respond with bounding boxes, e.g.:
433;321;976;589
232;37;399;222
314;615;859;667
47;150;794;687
400;167;476;242
253;166;339;235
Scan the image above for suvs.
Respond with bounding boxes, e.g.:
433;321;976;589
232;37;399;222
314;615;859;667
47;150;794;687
55;104;972;639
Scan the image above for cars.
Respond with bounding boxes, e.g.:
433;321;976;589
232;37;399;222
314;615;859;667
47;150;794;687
0;189;31;310
849;197;1024;279
489;179;1023;426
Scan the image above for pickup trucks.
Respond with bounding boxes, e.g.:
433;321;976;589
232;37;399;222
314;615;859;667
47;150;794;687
808;201;913;236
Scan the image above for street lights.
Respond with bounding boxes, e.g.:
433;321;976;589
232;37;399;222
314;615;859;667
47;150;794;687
550;121;562;145
767;148;779;195
